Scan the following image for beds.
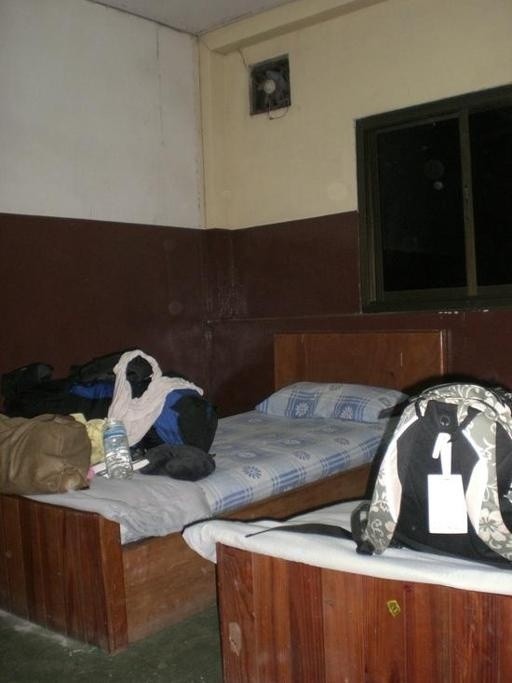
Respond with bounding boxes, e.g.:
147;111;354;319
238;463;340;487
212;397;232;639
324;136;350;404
213;479;512;683
1;324;450;655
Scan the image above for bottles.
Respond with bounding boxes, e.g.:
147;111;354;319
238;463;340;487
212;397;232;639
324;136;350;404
98;415;136;479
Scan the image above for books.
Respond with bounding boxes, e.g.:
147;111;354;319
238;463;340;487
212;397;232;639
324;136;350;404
90;455;111;473
100;446;150;481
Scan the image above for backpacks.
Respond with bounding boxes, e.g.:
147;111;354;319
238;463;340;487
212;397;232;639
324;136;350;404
351;376;512;575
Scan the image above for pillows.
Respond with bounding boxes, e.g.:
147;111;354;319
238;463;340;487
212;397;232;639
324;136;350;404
254;379;415;425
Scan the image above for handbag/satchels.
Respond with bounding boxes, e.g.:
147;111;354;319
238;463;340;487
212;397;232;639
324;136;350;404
0;346;220;494
